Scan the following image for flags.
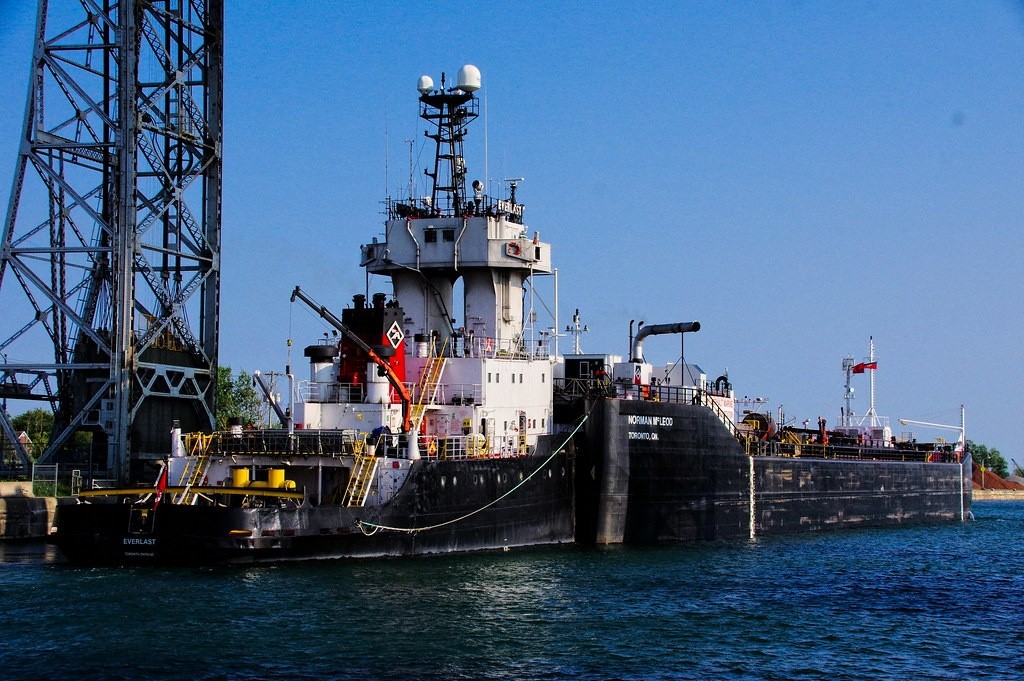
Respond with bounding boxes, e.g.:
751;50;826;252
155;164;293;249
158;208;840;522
852;362;878;373
153;470;166;509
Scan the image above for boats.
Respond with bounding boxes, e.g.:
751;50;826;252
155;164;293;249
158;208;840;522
60;65;972;567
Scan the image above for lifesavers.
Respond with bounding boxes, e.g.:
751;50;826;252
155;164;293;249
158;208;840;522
508;242;521;255
485;336;494;353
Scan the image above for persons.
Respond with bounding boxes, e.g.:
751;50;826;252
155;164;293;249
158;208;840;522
465;330;475;356
